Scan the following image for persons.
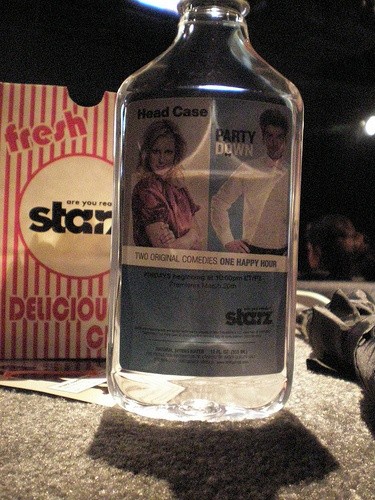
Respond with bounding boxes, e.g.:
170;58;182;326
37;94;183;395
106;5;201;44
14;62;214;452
131;121;206;251
211;110;291;256
300;213;372;282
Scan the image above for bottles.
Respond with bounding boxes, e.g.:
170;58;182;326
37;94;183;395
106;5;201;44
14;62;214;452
104;0;303;422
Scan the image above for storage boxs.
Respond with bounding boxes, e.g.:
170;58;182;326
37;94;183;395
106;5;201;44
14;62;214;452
0;79;114;360
